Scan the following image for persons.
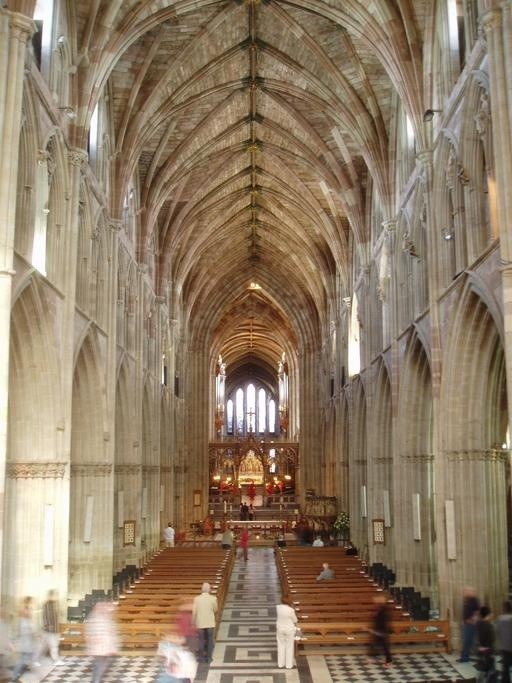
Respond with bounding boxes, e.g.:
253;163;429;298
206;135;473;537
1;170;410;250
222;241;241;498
325;533;338;547
155;630;200;683
363;592;394;670
315;561;336;582
493;599;512;682
0;587;67;682
454;583;482;664
273;534;287;556
274;593;300;669
237;528;252;561
162;521;176;548
345;541;358;555
174;596;200;663
222;524;234;550
312;534;325;548
239;501;256;521
85;599;124;683
474;607;496;682
190;581;219;663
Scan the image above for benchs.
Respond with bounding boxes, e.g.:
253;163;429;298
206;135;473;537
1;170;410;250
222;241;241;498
272;544;452;655
57;544;235;659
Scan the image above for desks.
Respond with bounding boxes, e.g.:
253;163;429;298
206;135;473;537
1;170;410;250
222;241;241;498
226;520;288;541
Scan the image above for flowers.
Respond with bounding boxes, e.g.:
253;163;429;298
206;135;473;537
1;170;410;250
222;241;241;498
331;511;351;538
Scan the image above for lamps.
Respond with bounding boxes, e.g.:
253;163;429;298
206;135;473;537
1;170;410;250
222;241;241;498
371;518;386;546
123;519;136;547
